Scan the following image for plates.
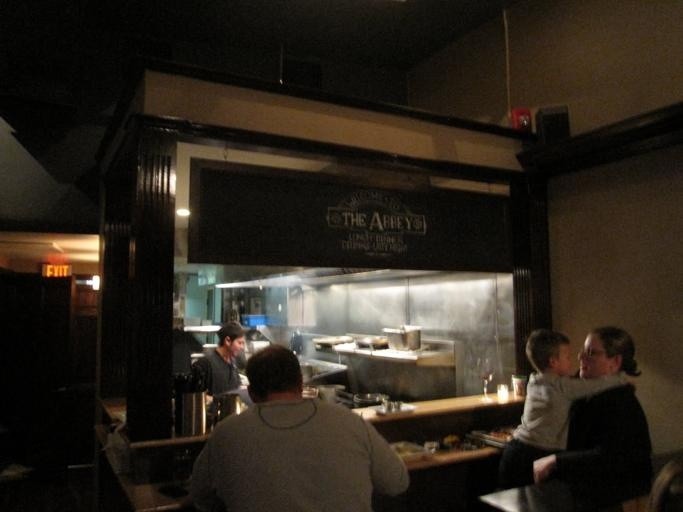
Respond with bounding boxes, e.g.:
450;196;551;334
373;403;415;415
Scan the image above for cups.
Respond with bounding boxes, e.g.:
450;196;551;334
512;374;527;395
497;383;509;401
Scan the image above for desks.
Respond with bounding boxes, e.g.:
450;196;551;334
477;450;682;512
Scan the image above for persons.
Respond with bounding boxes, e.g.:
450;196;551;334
497;328;638;491
533;326;654;511
190;322;245;429
188;343;411;512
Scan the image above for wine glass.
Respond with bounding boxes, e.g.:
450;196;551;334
477;357;495;403
424;440;436;455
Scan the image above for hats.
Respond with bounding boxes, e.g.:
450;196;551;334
217;322;250;336
